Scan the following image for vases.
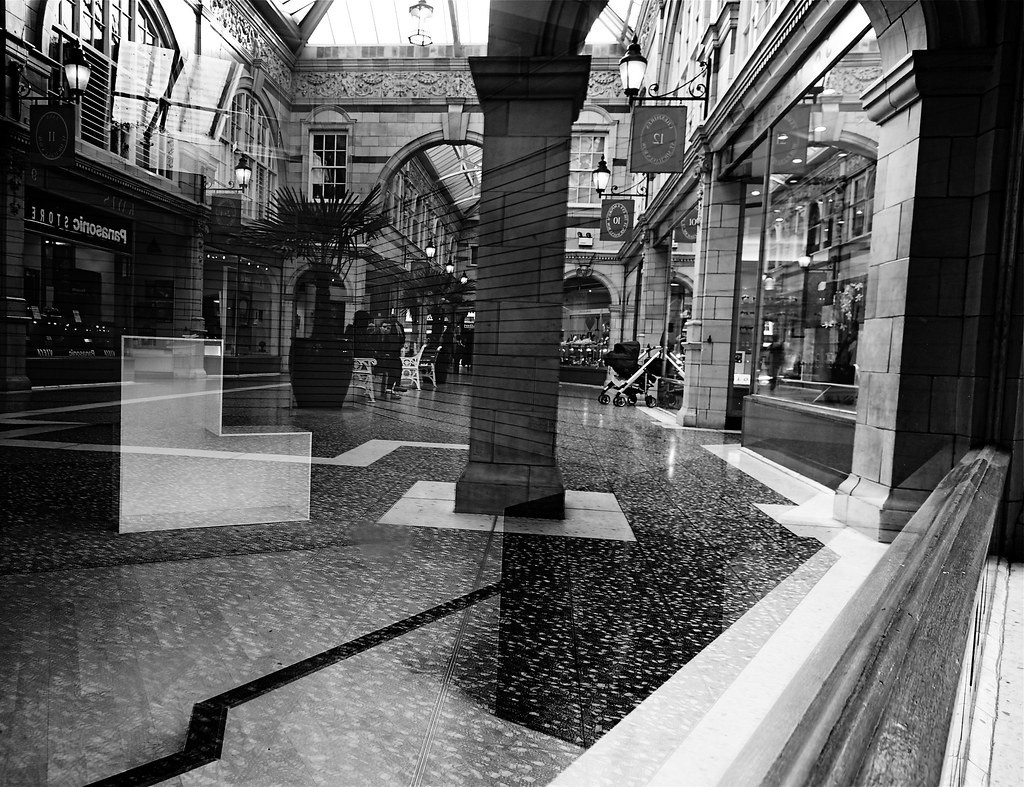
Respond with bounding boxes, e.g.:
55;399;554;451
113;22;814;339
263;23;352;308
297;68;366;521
820;363;855;385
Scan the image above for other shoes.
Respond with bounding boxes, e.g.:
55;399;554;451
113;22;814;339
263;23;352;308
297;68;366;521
387;393;401;400
393;385;407;392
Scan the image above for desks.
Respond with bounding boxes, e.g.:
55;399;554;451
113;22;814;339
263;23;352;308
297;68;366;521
404;347;441;390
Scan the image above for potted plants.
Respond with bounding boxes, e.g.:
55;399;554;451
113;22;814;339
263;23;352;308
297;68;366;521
227;182;392;409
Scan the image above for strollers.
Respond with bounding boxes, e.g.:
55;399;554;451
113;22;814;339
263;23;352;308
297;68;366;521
597;341;666;408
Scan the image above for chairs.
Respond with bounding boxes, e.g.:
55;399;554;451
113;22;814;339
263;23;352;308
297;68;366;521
349;358;377;403
383;344;442;395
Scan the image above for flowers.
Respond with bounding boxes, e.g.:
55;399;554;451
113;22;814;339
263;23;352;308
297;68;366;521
821;281;864;365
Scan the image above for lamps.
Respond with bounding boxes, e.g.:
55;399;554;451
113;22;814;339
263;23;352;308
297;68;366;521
618;33;712;107
404;238;436;262
577;232;593;246
407;0;434;48
11;39;92;105
796;249;836;272
591;154;649;198
459;270;468;284
201;154;252;193
437;256;454;273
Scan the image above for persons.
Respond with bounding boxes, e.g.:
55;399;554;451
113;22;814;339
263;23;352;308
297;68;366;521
345;311;408;400
834;319;859;385
461;330;474;372
765;334;786;391
442;325;455;343
